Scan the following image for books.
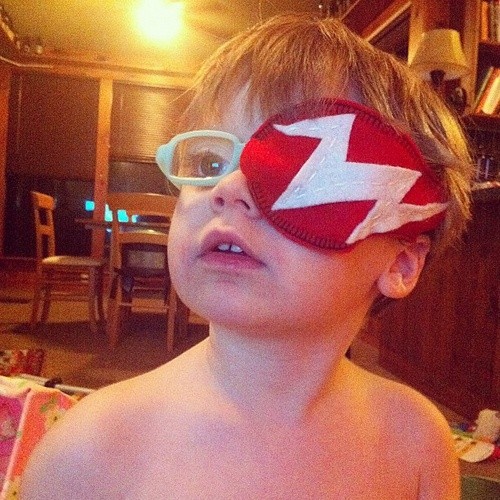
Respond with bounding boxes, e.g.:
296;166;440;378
477;0;500;44
474;64;500;116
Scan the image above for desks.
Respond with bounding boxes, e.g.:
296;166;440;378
74;218;205;338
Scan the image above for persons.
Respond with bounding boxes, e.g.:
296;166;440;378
22;10;474;500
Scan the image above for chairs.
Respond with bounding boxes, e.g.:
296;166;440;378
106;193;189;352
30;192;108;333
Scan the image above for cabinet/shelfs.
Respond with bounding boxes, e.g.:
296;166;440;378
318;0;500;207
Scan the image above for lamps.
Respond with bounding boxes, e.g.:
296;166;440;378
411;30;471;92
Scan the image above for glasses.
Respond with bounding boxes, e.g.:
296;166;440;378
155;130;246;192
